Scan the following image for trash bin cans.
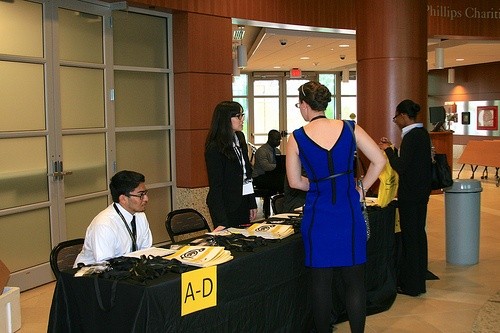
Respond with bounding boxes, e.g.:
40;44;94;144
441;179;483;266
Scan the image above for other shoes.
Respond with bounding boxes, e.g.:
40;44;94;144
397;287;420;297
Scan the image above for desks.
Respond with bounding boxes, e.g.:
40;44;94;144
47;194;402;333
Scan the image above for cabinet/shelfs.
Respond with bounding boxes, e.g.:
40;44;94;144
428;130;454;195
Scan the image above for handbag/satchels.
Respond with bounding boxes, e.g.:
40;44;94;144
361;210;370;241
430;149;453;191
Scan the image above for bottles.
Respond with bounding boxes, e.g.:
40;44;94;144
358;174;363;186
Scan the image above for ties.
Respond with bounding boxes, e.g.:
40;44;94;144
272;149;276;165
131;216;136;252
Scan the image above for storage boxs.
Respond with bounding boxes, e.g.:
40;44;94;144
0;287;22;333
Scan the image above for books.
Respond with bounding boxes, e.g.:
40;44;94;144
244;222;295;240
162;244;234;268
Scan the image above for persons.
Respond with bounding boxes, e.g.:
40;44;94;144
377;99;434;298
72;170;153;269
252;129;282;195
204;101;258;233
285;80;387;332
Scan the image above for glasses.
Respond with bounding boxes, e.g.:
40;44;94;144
126;191;149;200
393;113;402;123
295;103;302;108
231;114;244;120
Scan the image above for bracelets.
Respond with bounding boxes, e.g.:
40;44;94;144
358;185;368;193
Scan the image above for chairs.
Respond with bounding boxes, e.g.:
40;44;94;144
165;208;211;242
272;195;305;214
50;239;84;280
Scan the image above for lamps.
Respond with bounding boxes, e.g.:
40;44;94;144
435;43;444;69
232;24;247;76
342;67;350;83
448;67;455;83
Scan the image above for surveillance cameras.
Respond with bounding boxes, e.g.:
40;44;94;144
279;39;287;45
340;55;345;59
